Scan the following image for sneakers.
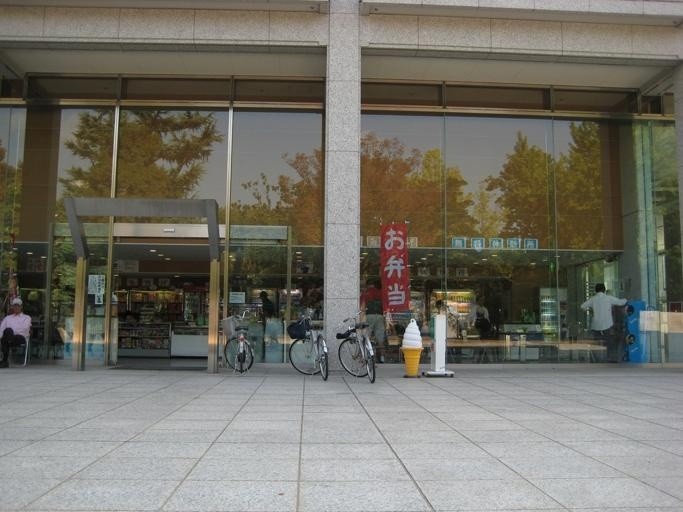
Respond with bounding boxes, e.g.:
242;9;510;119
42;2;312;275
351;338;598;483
0;360;9;369
609;359;621;363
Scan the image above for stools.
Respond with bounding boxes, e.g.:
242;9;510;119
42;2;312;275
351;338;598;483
7;334;30;368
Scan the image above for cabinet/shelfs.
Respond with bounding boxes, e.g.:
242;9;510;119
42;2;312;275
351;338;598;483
429;288;477;321
536;287;569;338
118;322;224;359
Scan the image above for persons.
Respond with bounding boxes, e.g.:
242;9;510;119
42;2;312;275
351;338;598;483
432;300;467;356
465;292;491;340
579;282;628;364
360;278;385;362
256;290;273;360
0;297;31;370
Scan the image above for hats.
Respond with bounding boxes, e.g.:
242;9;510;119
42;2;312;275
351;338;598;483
12;298;22;307
258;291;268;296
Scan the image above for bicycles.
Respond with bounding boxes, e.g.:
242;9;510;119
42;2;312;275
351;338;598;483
334;306;377;383
283;308;329;383
219;307;256;378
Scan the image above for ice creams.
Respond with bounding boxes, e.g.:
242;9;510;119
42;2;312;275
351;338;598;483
400;318;423;378
